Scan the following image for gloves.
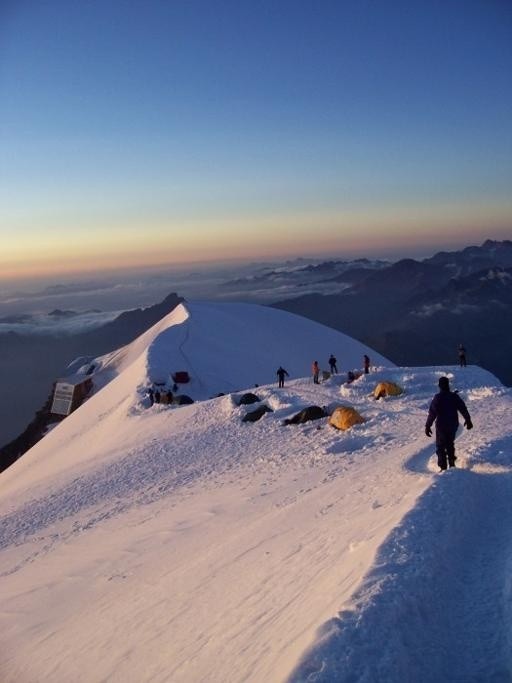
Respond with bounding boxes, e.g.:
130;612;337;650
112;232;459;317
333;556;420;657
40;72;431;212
464;418;473;429
425;425;432;437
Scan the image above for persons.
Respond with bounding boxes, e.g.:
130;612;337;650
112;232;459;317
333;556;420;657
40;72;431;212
457;342;468;367
329;354;338;374
275;365;289;387
363;353;370;374
148;383;178;406
424;376;473;473
312;361;320;384
